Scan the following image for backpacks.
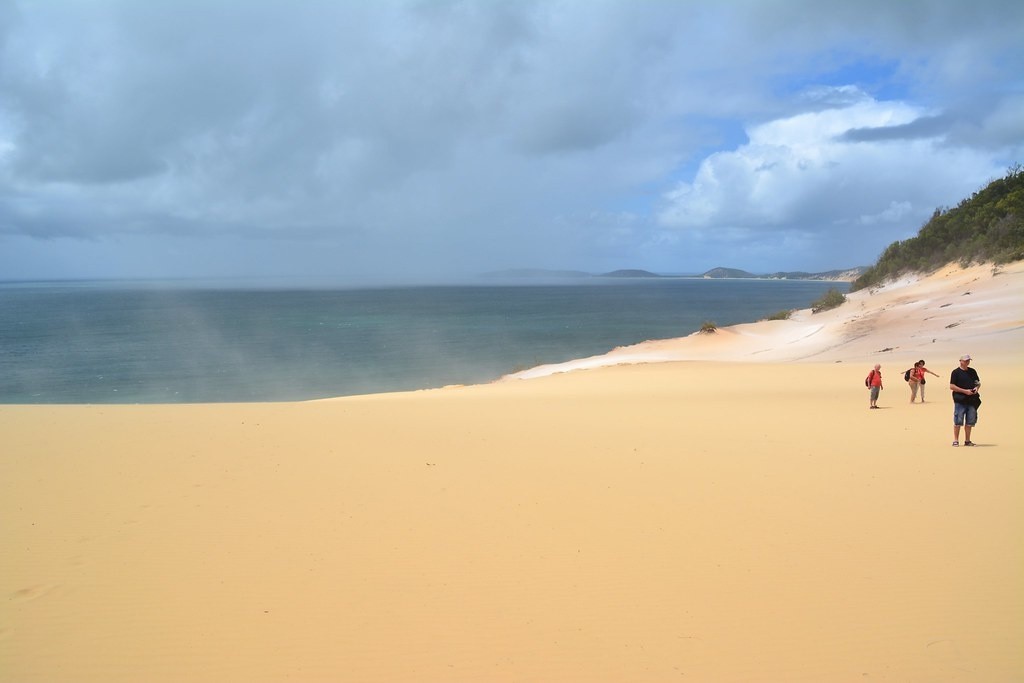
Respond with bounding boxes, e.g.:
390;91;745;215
864;369;882;387
904;367;916;382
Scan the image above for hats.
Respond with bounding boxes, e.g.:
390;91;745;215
959;354;972;360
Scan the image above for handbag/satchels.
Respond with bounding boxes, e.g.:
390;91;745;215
921;379;925;384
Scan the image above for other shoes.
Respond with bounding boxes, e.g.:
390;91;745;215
952;441;959;447
870;406;874;409
873;405;879;408
964;441;976;446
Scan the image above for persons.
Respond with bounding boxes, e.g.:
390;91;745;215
868;364;883;409
901;360;939;403
950;355;981;447
